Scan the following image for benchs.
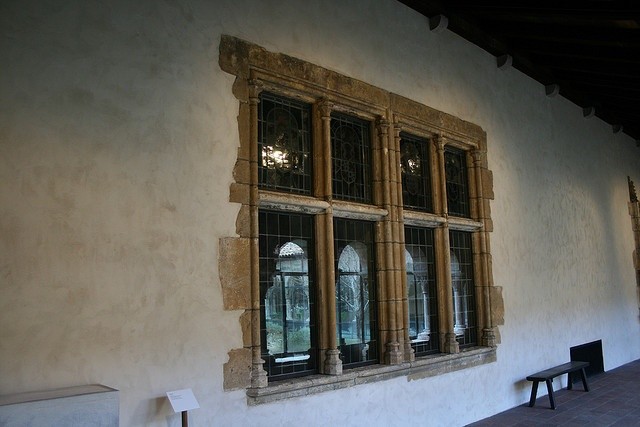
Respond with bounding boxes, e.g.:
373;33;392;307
526;361;590;410
